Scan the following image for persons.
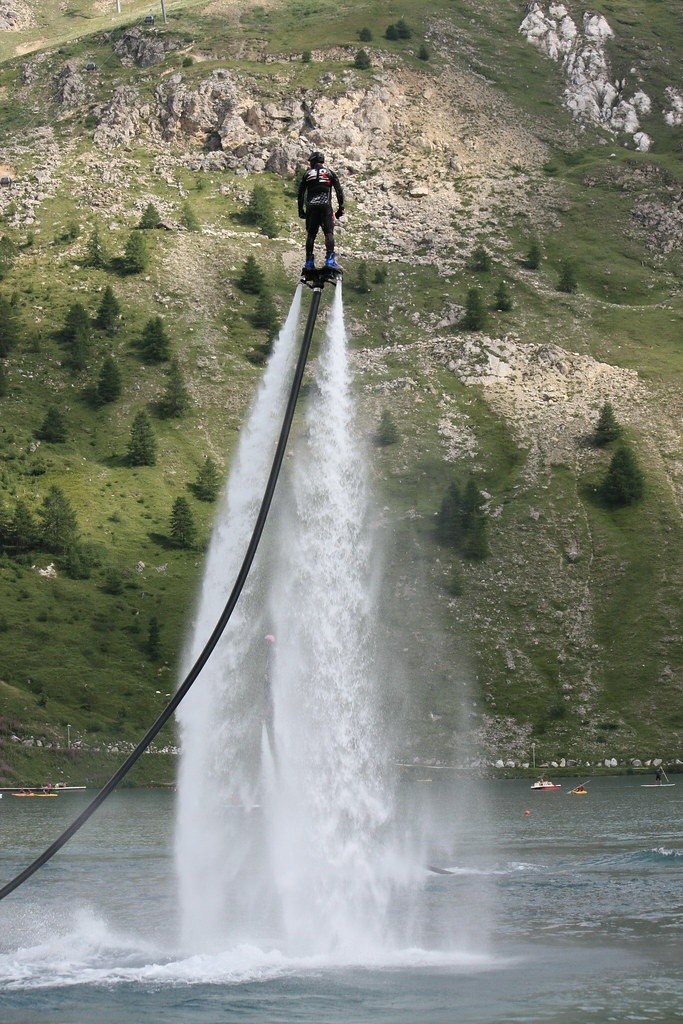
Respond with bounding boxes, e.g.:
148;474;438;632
297;151;346;274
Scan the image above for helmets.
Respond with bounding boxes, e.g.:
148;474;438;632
308;152;324;162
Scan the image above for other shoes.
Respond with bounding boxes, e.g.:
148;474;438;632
326;254;338;267
305;261;315;268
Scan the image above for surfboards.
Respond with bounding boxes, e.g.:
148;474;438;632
640;784;675;787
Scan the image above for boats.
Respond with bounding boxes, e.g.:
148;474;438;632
531;785;562;790
640;783;677;788
571;790;588;795
10;792;59;798
416;780;433;782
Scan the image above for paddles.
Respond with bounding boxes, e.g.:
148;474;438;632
565;780;591;795
660;766;669;782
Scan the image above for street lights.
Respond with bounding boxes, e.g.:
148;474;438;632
67;723;72;749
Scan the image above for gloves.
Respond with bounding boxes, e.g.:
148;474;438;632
298;210;306;219
336;206;345;218
